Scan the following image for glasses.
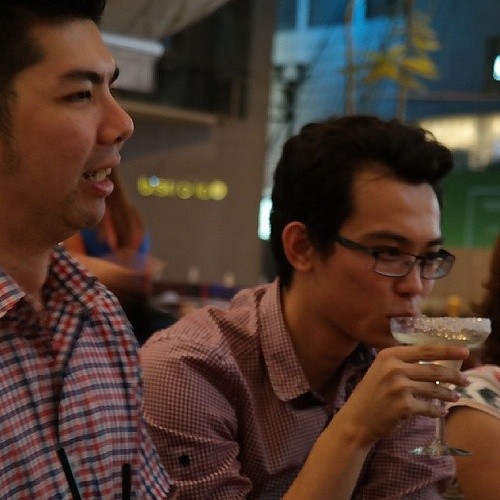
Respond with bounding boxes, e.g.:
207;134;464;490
279;216;456;280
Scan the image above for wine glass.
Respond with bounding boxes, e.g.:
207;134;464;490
390;317;491;456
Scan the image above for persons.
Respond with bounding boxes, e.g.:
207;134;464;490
0;0;182;500
441;232;500;500
62;148;167;350
146;116;470;500
